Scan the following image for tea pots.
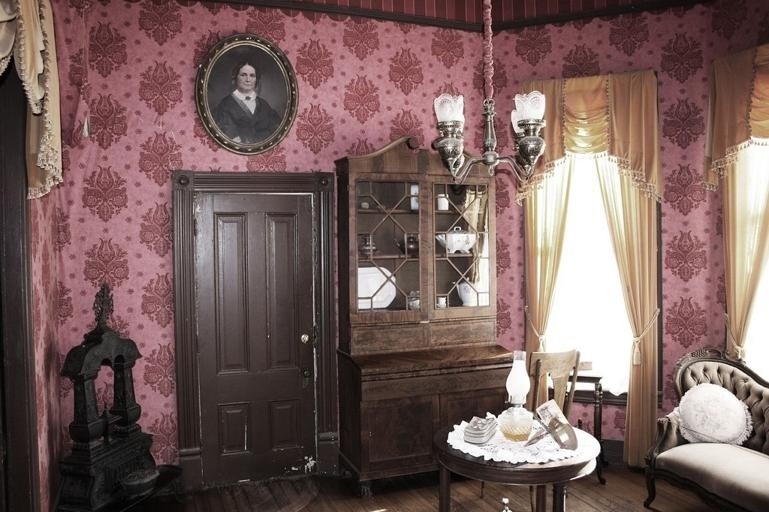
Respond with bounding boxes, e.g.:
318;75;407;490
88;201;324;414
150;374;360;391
451;275;481;306
393;232;420;257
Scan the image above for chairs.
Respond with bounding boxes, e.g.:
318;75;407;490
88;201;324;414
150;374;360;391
480;348;581;512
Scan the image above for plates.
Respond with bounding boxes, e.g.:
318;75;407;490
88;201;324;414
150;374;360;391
358;266;398;312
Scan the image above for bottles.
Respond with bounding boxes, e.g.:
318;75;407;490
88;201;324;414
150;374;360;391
551;418;579;450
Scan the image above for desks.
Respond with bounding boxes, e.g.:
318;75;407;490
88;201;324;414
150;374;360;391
431;417;602;512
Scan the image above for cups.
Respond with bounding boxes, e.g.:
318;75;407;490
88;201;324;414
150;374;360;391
437;193;450;210
437;297;446;308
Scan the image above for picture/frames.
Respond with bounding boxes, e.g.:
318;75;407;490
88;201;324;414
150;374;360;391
197;31;299;154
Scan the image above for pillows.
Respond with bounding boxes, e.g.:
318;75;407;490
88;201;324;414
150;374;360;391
672;382;752;446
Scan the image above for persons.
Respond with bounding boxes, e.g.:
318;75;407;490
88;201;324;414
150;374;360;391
210;62;282;147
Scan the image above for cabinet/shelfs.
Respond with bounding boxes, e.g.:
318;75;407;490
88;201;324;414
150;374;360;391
338;342;518;498
336;134;497;356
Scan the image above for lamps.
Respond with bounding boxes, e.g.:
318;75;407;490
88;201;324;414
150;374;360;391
426;0;546;185
504;351;530;410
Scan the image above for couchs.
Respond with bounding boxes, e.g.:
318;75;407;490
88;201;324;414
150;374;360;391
644;346;769;511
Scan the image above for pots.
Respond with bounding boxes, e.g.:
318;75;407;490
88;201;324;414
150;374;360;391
434;225;481;254
